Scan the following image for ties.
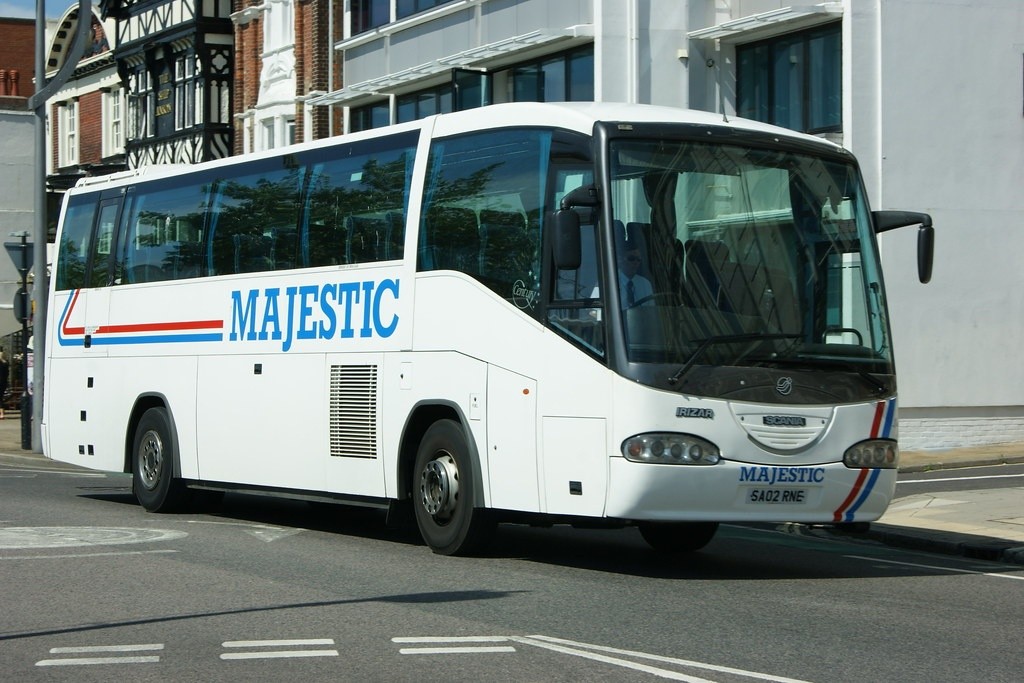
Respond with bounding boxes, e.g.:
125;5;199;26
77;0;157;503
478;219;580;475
627;281;635;306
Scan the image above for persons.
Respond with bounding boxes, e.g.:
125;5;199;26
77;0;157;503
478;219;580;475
0;346;10;418
350;225;402;265
586;240;655;322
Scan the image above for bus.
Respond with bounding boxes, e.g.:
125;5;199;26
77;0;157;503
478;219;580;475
40;101;938;558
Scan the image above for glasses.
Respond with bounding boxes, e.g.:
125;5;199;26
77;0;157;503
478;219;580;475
622;255;641;261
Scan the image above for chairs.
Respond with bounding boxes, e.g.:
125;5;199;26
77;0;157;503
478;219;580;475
77;206;803;332
